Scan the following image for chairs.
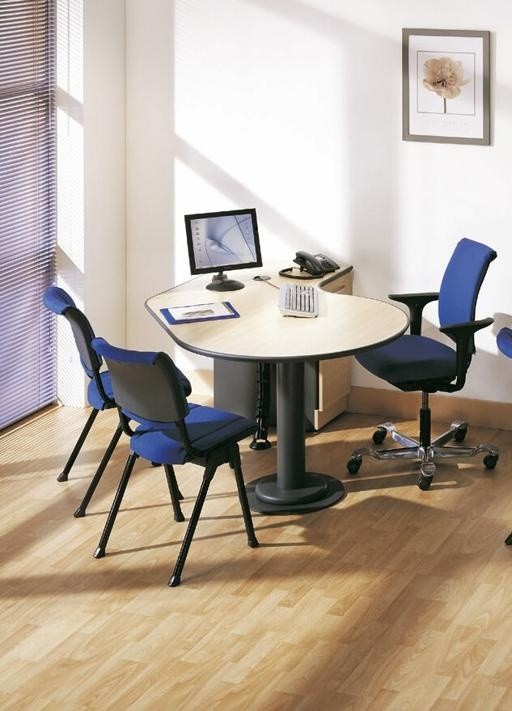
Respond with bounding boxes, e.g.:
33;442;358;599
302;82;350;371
88;337;261;587
41;286;192;519
346;238;499;491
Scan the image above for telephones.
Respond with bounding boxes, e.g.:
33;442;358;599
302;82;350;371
294;251;340;275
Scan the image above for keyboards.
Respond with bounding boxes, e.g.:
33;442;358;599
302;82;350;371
279;283;319;318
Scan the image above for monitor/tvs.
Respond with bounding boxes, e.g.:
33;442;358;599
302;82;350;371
184;208;263;292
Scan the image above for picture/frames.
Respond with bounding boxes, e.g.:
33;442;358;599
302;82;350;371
400;27;490;146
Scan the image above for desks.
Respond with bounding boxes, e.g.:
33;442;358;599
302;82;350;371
145;263;411;516
